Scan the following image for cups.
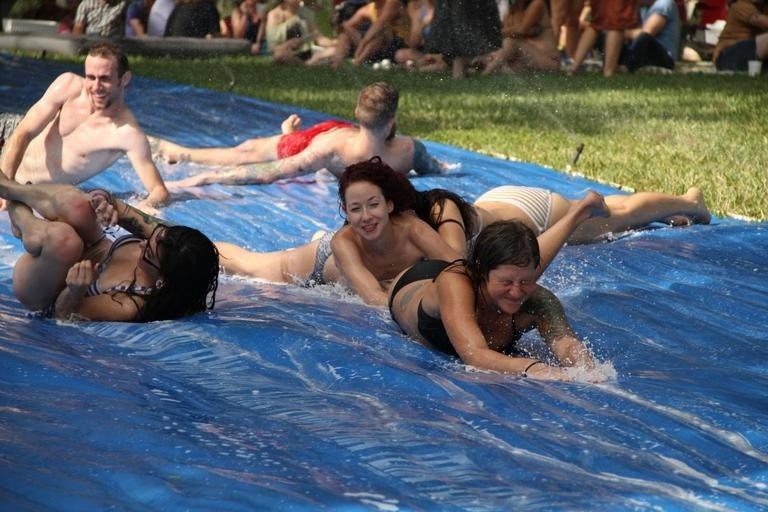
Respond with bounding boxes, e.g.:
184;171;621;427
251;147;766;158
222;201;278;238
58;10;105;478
747;60;762;79
696;61;717;75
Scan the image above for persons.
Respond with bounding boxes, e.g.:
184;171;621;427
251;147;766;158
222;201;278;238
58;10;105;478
414;186;712;250
0;44;171;211
1;173;221;324
389;219;595;384
73;0;768;77
153;80;440;192
215;156;460;307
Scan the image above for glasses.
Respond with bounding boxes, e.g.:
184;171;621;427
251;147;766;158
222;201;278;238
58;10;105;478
142;223;167;274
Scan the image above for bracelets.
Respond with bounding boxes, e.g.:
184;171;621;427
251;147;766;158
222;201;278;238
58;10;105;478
525;361;543;373
